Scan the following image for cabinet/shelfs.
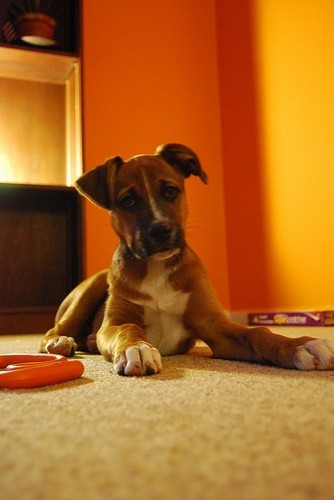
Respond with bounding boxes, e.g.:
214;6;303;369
0;0;82;336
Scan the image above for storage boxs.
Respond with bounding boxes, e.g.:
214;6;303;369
248;310;334;325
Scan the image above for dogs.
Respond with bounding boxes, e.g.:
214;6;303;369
37;143;333;378
